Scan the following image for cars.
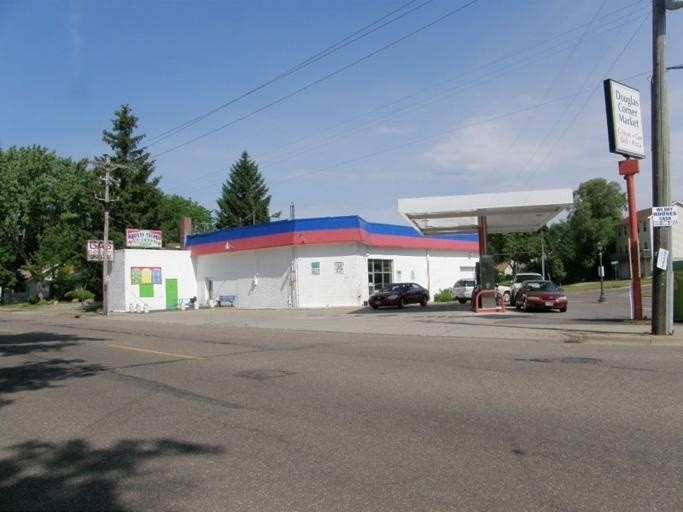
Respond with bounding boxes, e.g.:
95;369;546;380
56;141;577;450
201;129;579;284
453;272;568;312
368;283;429;309
